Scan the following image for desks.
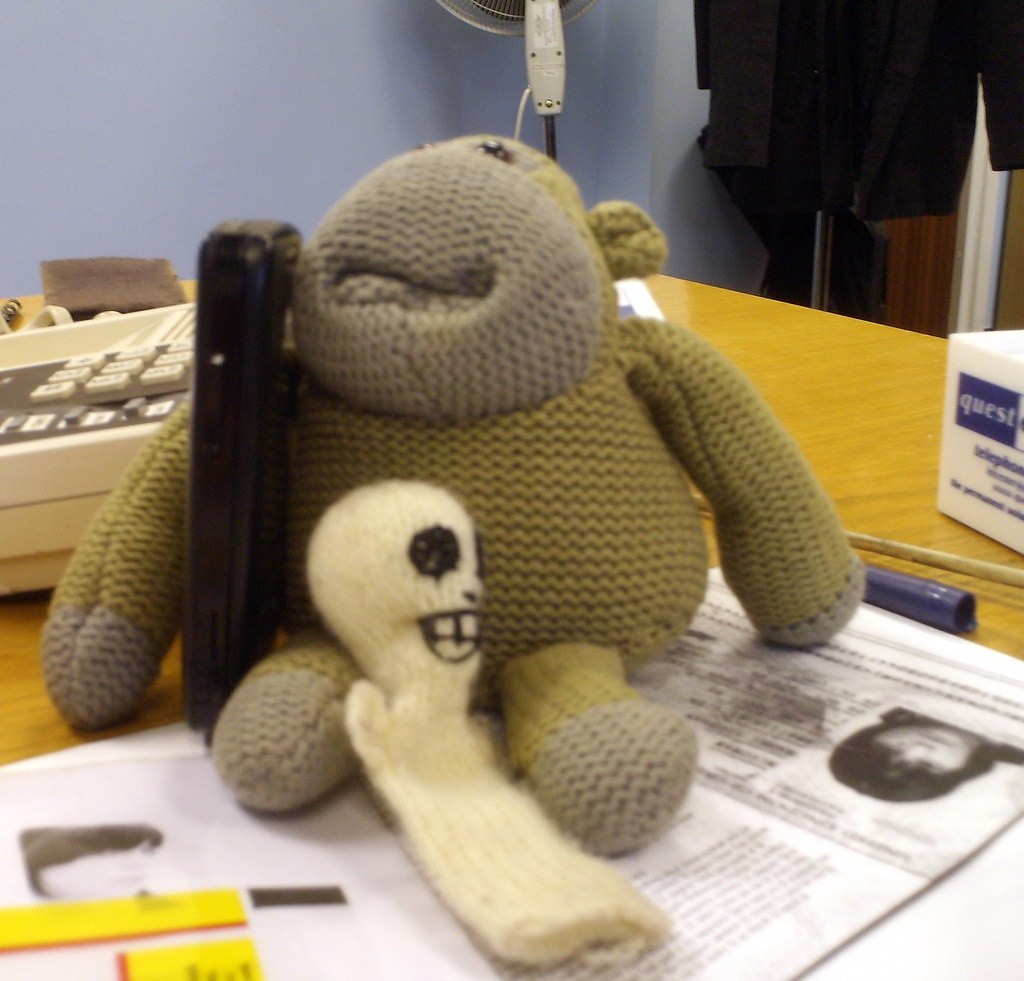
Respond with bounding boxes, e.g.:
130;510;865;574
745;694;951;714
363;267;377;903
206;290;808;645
0;268;1024;981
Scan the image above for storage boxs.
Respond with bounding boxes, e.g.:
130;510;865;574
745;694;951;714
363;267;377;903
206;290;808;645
936;329;1024;556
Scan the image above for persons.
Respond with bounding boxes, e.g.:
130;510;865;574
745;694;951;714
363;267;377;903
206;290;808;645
831;708;1024;802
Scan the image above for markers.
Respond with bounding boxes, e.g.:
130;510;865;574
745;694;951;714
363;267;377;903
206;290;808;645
863;562;979;636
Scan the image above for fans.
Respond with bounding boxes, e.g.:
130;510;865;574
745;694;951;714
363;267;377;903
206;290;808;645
437;0;599;163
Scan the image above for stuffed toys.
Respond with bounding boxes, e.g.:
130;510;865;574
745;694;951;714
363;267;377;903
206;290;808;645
41;133;860;846
309;481;661;966
